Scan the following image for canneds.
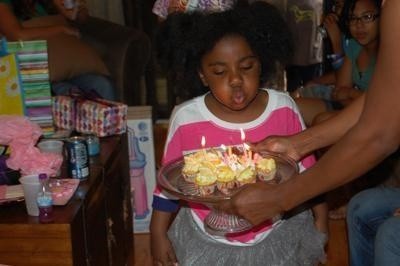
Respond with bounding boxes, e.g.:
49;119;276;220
63;137;90;182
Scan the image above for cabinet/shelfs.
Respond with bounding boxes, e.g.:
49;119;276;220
0;131;135;266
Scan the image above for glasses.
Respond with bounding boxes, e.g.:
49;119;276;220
347;13;380;25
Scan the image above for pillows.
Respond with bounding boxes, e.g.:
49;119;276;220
21;13;111;82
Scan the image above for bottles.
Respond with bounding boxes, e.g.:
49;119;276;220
317;4;342;36
36;174;54;223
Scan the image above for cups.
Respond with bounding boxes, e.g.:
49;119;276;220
38;141;64;177
18;175;41;217
81;131;100;156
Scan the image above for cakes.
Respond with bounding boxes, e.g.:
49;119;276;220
181;146;278;196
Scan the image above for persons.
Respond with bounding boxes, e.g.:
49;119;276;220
147;0;399;266
0;0;115;100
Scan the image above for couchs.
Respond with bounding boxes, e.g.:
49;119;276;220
0;15;152;106
